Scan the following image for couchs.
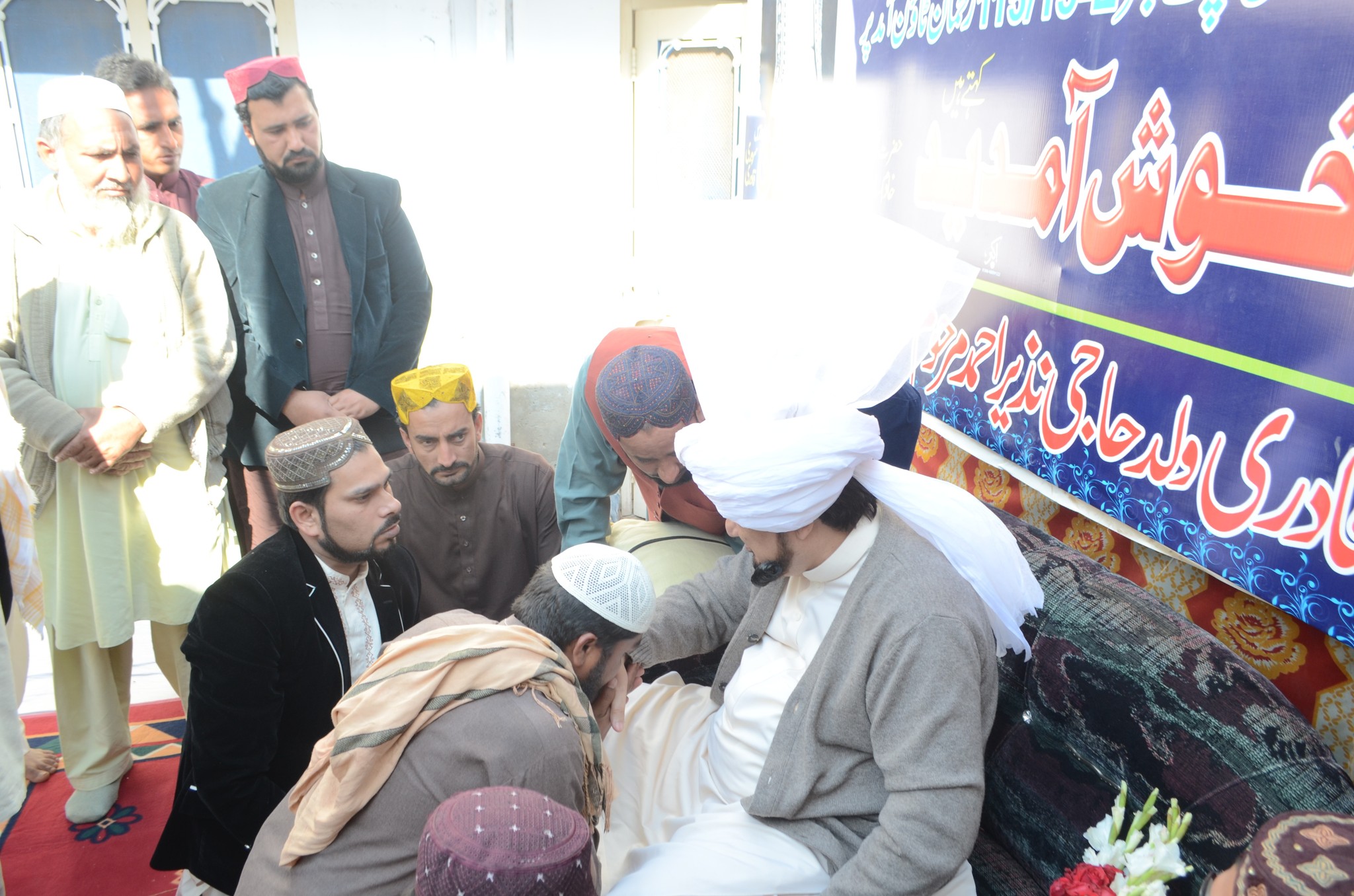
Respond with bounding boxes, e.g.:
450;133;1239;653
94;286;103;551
648;500;1354;894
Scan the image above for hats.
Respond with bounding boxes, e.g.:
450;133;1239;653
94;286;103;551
391;363;478;423
672;401;887;533
36;71;131;121
412;782;602;895
265;415;371;492
550;540;654;635
595;345;694;437
226;54;306;104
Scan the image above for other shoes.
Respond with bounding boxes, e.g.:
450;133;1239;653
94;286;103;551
64;774;122;823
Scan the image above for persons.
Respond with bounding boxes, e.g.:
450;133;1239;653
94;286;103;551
409;784;604;896
594;404;1001;896
92;52;218;223
148;415;424;896
0;385;60;785
232;541;657;896
553;319;727;555
382;363;564;631
1;70;242;824
194;55;435;558
